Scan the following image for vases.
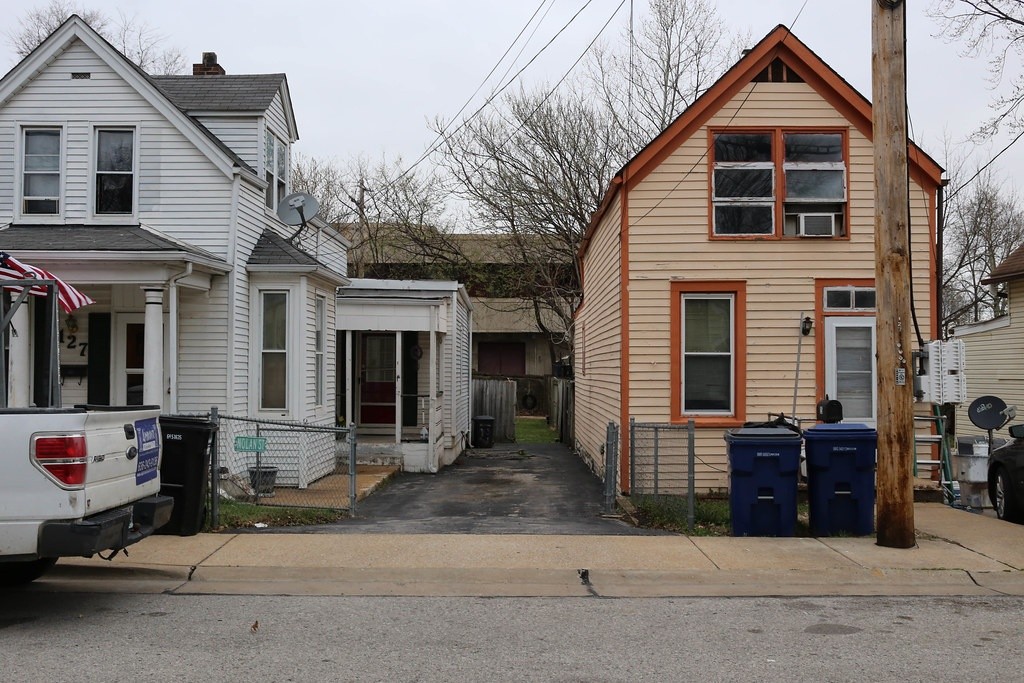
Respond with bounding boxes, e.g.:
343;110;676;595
249;466;279;498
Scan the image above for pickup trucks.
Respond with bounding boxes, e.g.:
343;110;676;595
0;271;174;594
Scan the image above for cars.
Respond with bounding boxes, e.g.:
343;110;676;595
987;424;1024;525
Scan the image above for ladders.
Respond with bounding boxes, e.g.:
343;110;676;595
914;405;956;504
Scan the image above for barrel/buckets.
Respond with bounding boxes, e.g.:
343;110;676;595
249;465;279;494
973;444;989;456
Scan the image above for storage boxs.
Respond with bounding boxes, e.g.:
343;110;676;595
953;454;1003;508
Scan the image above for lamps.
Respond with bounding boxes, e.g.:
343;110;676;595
802;318;812;335
66;315;78;333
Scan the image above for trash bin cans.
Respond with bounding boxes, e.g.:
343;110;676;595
472;416;496;448
723;428;803;537
801;423;878;537
150;415;219;537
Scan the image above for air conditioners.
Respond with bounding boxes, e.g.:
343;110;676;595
796;215;835;237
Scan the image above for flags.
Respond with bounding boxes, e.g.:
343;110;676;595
0;248;95;314
